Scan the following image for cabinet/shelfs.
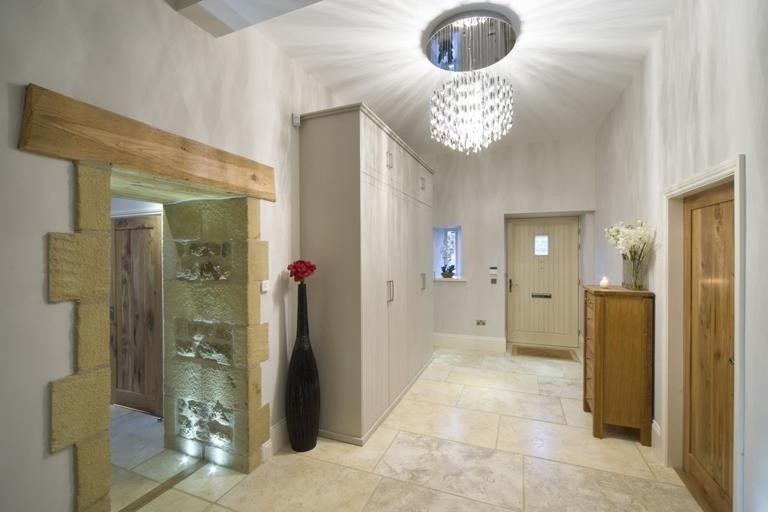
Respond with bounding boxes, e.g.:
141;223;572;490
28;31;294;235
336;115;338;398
301;102;436;447
583;284;655;446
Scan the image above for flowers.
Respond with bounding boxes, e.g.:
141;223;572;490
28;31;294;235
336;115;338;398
607;220;649;289
287;260;317;285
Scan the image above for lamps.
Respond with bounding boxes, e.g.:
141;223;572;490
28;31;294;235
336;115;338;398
419;2;521;156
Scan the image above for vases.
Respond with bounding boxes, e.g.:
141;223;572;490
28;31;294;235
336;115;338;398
286;283;321;452
622;253;648;291
